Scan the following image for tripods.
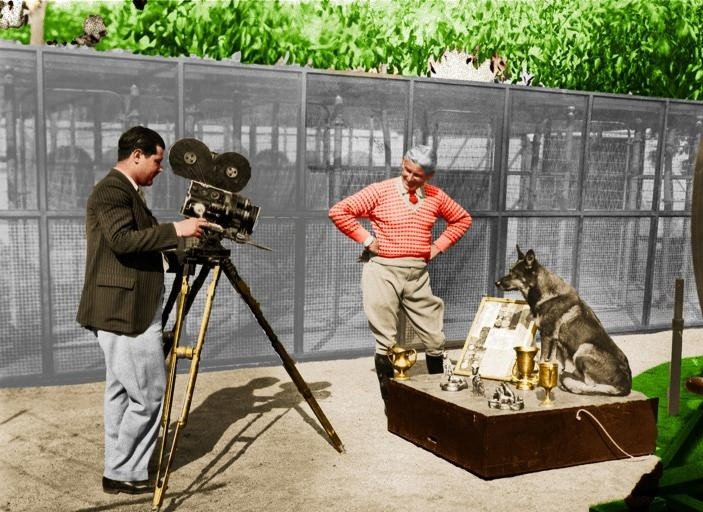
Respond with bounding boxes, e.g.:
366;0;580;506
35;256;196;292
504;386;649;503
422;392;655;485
151;249;343;511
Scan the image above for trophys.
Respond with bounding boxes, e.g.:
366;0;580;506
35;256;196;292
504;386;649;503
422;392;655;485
510;344;539;392
536;358;558;406
385;344;416;382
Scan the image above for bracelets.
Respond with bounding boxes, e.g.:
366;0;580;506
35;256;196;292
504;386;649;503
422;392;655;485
362;236;375;247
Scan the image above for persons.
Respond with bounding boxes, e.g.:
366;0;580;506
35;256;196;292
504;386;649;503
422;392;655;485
75;125;213;496
325;140;472;421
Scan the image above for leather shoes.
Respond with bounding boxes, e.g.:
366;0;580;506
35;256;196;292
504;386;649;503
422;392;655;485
102;475;152;493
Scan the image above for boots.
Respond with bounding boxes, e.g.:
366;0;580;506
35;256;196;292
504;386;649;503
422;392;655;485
425;353;445;374
374;352;395;416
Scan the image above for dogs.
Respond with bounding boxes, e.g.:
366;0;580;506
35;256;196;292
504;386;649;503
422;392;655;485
492;244;633;397
